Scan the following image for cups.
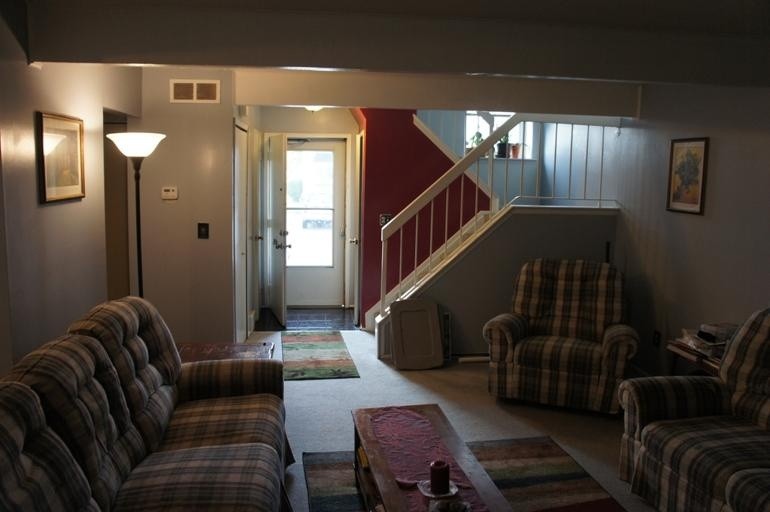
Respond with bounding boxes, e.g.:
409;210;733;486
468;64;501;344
430;460;449;496
511;144;520;158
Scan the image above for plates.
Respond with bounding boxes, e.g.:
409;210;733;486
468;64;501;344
416;479;458;498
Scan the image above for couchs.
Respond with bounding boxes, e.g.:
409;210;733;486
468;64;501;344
616;308;770;511
484;253;636;417
0;296;295;511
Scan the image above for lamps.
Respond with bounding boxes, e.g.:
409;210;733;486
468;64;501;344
104;133;169;299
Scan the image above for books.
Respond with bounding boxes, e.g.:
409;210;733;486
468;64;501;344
666;322;739;370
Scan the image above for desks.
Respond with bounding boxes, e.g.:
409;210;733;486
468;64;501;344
665;330;737;376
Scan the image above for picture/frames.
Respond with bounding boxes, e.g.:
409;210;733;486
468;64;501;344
34;113;86;203
663;138;708;214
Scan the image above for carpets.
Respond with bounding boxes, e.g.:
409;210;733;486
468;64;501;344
278;327;359;381
304;436;628;512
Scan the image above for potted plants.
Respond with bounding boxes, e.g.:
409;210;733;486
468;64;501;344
467;131;520;158
